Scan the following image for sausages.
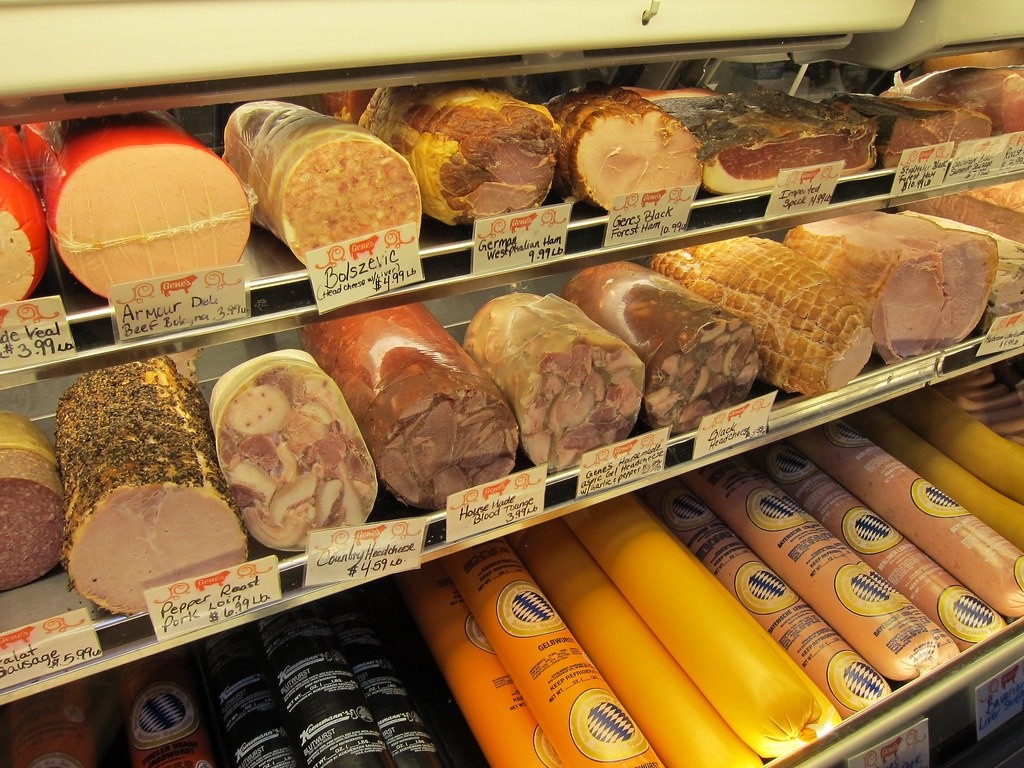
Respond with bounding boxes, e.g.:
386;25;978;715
197;394;1024;767
